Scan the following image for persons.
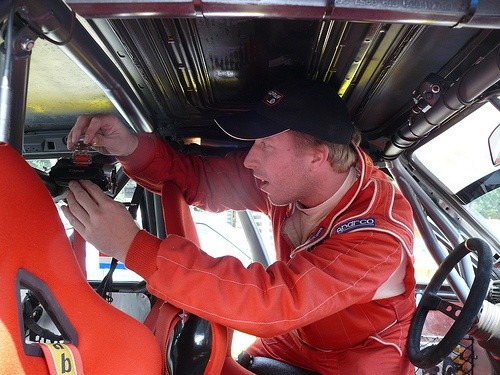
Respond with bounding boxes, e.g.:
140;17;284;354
61;83;418;375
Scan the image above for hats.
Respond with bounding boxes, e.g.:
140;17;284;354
212;77;352;145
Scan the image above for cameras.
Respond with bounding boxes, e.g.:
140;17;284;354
41;139;117;198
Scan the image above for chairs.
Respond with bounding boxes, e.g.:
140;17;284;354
1;140;164;375
134;180;256;374
